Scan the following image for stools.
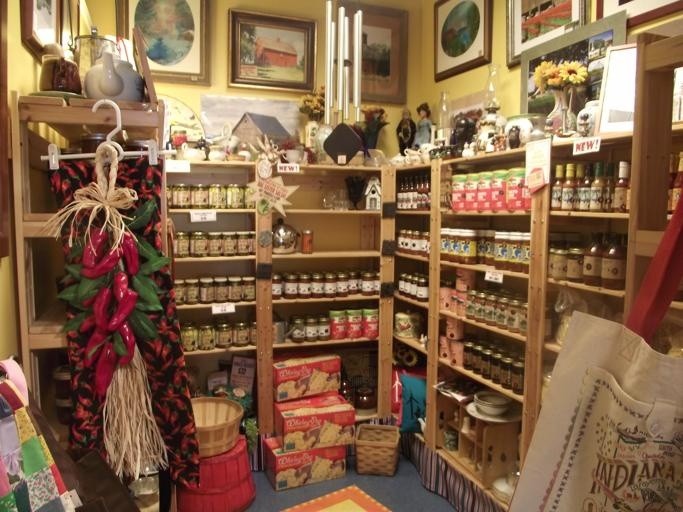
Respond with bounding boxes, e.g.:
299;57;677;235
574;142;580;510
173;433;256;510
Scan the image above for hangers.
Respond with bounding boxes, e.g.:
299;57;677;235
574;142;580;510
38;97;171;170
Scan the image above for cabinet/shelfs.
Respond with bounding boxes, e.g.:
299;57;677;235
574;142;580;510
395;137;631;511
169;160;382;471
623;34;683;355
11;90;176;510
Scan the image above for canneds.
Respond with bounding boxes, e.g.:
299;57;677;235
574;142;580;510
165;183;258;209
289;317;329;342
173;232;255;256
131;138;156;157
174;278;256;305
181;321;256;352
547;242;583;282
79;133;106;153
56;400;76;425
270;272;378;297
356;388;374;409
397;228;431;257
440;228;530;395
398;271;429;303
52;372;74;400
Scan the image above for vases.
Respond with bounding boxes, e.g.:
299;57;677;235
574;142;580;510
546;89;577;132
305;120;320;146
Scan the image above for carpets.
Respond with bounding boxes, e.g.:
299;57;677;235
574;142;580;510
283;484;391;511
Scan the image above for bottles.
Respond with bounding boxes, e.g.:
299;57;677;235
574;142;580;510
550;150;683;214
437;90;451;141
340;373;353;408
582;232;626;289
396;174;430;211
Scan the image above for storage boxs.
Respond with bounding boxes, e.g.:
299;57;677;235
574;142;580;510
263;436;346;492
272;352;342;402
187;397;241;459
271;392;354;452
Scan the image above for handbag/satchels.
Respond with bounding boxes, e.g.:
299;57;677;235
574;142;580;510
507;312;683;511
0;358;141;512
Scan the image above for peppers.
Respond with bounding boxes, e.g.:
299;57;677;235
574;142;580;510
78;227;140;401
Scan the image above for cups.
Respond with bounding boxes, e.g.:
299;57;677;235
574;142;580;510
333;189;348;212
403;145;435;166
281;150;299;164
301;229;312;253
319;185;337;209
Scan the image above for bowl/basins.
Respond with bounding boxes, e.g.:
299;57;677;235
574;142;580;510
473;392;511;416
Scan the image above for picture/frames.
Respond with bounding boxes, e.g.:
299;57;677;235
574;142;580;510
20;0;61;64
597;0;683;28
520;9;627;116
336;0;408;106
505;0;592;66
434;0;494;82
595;43;683;135
228;8;317;93
116;0;210;85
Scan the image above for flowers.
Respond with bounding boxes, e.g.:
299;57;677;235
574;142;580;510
534;60;587;95
299;86;325;120
351;108;389;157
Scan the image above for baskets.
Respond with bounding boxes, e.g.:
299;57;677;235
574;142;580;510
354;423;401;476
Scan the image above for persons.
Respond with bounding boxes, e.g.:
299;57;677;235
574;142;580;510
412;100;431;148
395;108;414;154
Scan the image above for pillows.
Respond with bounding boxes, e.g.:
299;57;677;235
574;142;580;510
398;373;426;434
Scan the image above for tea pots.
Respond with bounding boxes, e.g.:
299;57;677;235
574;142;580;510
38;54;82;94
83;49;142;102
271;218;301;253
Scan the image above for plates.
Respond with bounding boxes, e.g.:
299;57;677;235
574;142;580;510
465;401;523;422
